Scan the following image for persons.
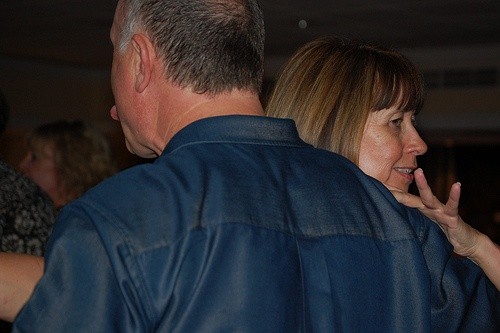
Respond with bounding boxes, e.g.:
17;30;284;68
0;91;61;333
0;38;500;333
12;0;500;333
18;119;117;212
